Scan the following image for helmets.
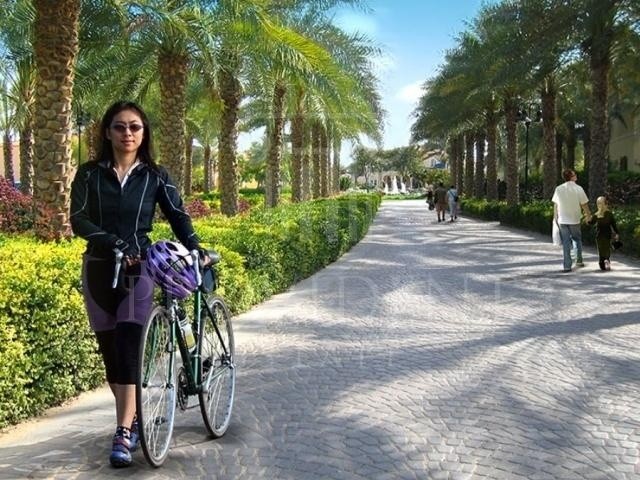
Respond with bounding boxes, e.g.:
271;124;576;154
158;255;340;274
146;240;198;299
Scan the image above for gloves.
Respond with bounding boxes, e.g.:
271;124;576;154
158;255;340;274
113;238;138;266
196;245;211;267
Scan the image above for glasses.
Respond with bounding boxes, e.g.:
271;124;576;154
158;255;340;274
111;123;144;132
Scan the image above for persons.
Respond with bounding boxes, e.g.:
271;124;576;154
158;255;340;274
551;169;592;271
425;182;460;222
586;196;619;270
70;101;210;465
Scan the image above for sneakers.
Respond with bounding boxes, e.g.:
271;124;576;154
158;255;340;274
128;421;140;452
563;259;610;273
109;431;133;468
438;217;457;223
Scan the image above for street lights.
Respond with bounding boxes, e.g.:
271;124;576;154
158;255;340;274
513;108;544;207
75;110;94;167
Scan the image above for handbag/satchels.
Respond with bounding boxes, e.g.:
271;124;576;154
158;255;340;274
454;196;459;202
552;218;562;246
434;197;439;204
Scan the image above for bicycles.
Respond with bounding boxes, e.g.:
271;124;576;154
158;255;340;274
106;247;238;467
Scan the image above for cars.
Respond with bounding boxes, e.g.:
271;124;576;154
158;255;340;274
13;181;34;196
